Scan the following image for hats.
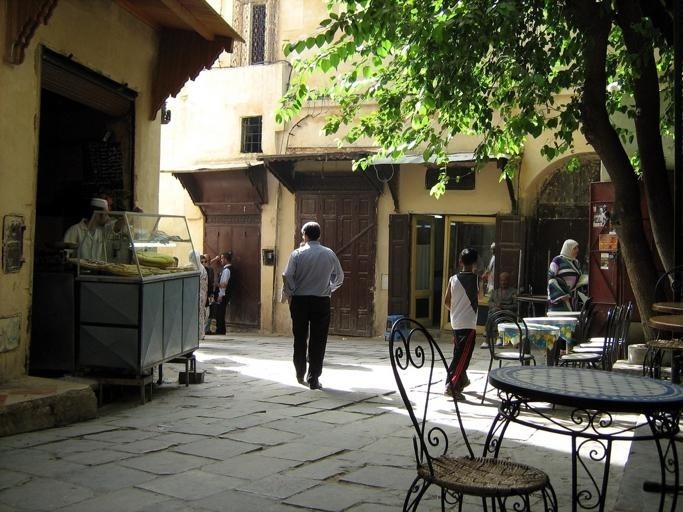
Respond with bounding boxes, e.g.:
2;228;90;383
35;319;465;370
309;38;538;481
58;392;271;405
90;198;108;209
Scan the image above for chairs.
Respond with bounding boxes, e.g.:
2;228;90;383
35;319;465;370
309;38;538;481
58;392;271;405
390;317;557;512
480;298;633;403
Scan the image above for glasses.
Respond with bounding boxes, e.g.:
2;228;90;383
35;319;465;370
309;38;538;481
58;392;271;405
201;261;207;263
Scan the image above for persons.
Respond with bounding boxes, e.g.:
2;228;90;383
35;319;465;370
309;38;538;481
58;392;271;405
445;248;479;402
480;272;517;350
62;189;146;264
482;239;496;302
172;249;233;340
281;221;344;389
546;239;585;312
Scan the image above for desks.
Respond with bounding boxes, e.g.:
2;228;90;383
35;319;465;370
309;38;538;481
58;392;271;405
481;365;682;512
643;301;683;385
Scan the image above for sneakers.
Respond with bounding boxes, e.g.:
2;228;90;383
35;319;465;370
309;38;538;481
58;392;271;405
496;338;503;345
461;379;470;390
443;383;465;401
480;342;489;348
216;327;226;334
210;319;217;333
205;330;216;334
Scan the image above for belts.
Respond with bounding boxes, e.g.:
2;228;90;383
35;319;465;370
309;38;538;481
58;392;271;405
293;296;331;302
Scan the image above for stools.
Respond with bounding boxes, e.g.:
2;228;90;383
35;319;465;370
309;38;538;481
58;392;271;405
385;315;408;342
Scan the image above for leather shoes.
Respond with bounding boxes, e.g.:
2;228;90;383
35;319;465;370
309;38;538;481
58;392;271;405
296;375;304;383
309;382;322;389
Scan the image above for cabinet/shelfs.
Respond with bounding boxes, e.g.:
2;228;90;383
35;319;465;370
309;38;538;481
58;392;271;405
75;212;201;372
